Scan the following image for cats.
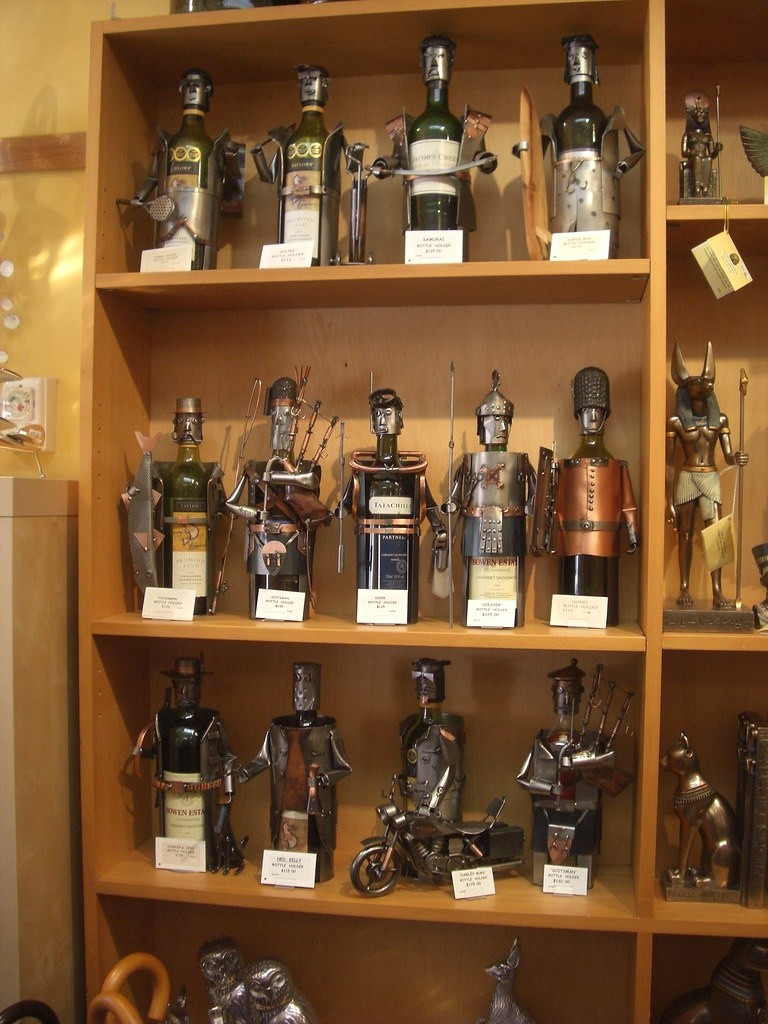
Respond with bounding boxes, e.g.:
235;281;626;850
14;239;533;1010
659;730;742;888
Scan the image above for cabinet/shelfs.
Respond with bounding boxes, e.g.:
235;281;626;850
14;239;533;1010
76;0;768;1024
0;476;85;1024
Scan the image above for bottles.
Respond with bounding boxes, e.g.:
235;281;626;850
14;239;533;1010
270;711;334;884
402;707;455;880
560;436;619;628
249;451;309;621
283;103;331;267
162;705;216;870
156;106;214;271
408;81;463;231
360;436;417;624
462;444;522;629
558;82;609;160
544;715;593;890
166;444;213;615
